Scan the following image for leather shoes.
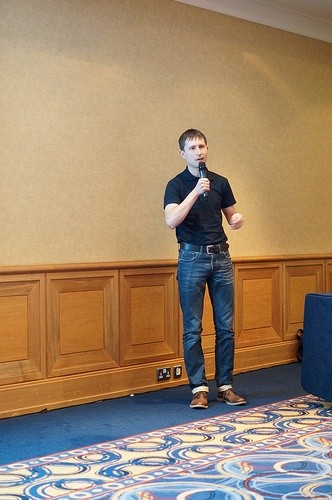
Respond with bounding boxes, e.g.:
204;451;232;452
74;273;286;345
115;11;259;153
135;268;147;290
217;389;246;406
190;392;209;408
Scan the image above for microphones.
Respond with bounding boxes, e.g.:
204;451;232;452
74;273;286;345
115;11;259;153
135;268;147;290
200;162;208;198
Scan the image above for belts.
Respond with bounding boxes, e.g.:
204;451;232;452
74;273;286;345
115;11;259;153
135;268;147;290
180;242;229;255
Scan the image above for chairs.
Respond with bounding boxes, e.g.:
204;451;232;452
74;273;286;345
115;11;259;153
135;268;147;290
300;292;332;402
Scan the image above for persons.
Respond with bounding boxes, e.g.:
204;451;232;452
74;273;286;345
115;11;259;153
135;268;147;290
164;130;246;408
